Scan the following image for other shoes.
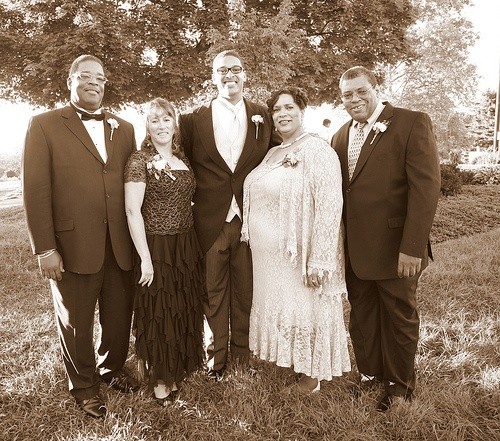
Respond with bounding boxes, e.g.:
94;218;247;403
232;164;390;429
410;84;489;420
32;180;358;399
153;387;178;406
207;364;227;379
281;376;320;395
376;395;411;412
353;378;375;398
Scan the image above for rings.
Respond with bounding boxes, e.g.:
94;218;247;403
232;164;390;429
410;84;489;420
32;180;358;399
312;278;317;282
145;277;151;281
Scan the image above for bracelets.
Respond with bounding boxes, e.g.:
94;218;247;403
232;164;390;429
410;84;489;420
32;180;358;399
38;248;57;260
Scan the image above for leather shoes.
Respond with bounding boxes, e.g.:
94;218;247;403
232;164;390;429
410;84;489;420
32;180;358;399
76;395;106;418
105;368;141;393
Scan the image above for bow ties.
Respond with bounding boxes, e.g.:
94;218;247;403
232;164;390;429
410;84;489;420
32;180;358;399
70;101;105;121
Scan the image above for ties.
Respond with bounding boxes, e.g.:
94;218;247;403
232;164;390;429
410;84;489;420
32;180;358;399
348;122;369;181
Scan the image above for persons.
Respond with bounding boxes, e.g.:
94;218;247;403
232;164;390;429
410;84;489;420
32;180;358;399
124;96;206;406
23;55;141;418
240;85;352;396
332;66;441;412
316;119;335;146
177;49;283;380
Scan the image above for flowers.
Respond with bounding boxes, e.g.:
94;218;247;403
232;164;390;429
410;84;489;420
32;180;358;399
370;121;390;144
107;118;119;140
251;115;263;140
146;154;167;175
284;146;302;167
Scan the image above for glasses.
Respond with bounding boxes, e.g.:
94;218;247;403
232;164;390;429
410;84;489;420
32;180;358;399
340;84;375;104
70;73;109;85
215;65;245;75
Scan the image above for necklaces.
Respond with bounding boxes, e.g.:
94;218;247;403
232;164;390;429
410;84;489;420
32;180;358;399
162;152;174;158
280;132;309;149
